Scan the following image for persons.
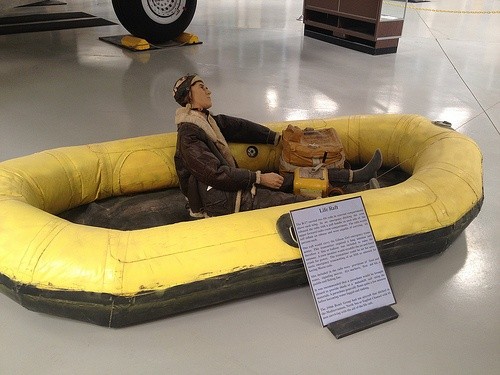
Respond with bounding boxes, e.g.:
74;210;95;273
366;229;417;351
172;73;382;218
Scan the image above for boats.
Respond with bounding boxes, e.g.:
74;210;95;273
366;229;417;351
0;115;486;329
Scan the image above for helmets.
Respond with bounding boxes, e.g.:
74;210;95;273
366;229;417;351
173;74;205;107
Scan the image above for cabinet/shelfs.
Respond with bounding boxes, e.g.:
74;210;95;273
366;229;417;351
302;0;408;56
